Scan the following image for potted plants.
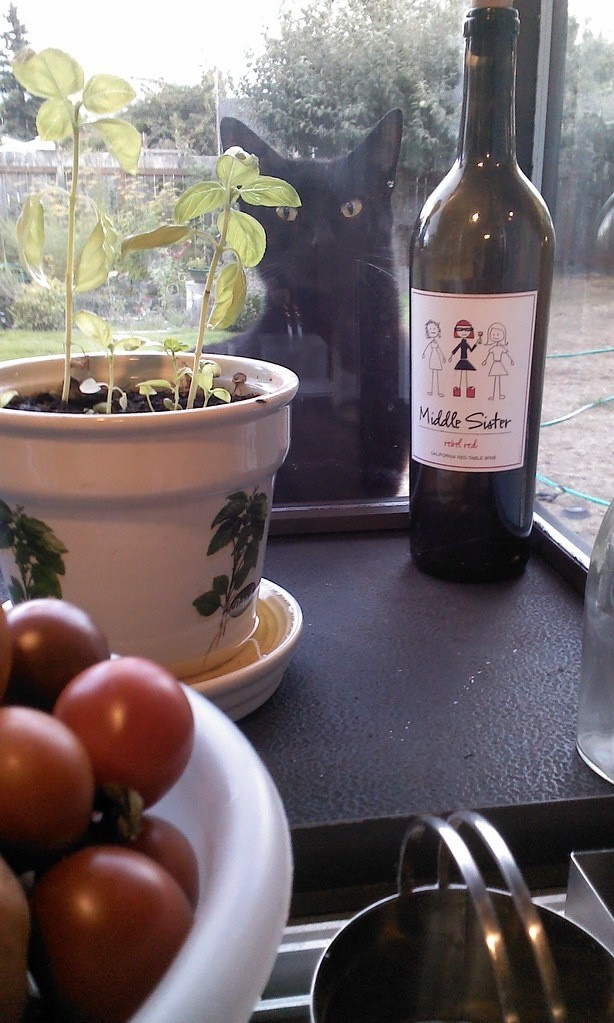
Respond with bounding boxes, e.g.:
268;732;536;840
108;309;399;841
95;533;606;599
0;42;303;676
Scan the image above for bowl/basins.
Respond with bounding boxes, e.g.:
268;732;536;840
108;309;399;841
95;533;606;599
109;650;295;1023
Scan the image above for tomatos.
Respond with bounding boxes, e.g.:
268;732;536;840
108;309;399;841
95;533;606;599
0;599;201;1023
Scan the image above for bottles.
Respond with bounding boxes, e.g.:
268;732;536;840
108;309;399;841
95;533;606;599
576;497;614;785
408;0;557;582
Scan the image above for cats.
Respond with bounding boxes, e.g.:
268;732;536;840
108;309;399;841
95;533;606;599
183;107;411;504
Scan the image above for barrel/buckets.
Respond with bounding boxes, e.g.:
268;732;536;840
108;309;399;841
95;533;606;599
307;807;614;1023
